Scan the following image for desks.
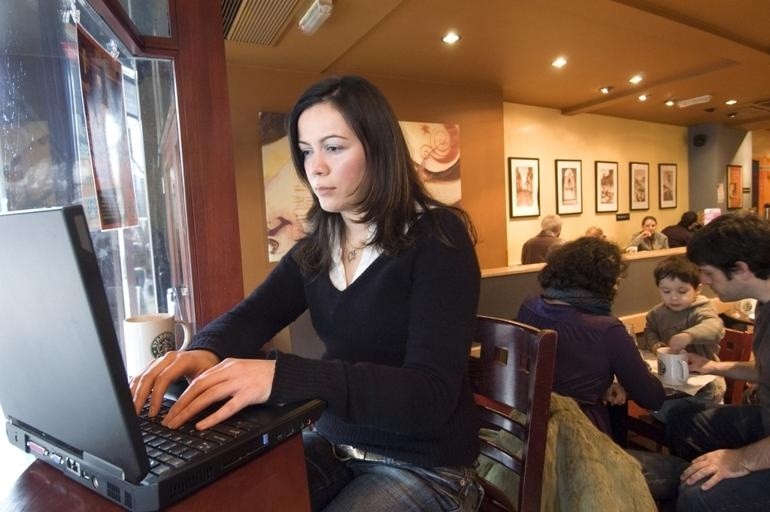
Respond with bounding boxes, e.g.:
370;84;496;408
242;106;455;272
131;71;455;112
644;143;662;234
5;321;312;512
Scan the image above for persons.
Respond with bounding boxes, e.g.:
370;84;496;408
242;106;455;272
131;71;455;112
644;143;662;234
585;226;604;240
658;210;770;510
127;77;480;512
512;237;690;499
622;216;669;251
687;222;703;233
4;120;60;209
521;214;562;263
661;212;698;247
645;256;724;404
90;222;154;316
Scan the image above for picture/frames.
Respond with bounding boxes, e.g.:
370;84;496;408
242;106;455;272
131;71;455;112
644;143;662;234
726;164;745;212
555;159;583;216
658;163;677;210
508;157;540;219
629;162;649;211
594;160;619;213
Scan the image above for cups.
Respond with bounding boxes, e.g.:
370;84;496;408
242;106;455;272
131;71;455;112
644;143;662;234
736;299;759;315
658;347;688;385
625;247;638;256
125;315;190;383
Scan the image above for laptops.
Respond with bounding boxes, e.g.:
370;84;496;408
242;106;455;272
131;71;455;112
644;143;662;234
0;205;325;512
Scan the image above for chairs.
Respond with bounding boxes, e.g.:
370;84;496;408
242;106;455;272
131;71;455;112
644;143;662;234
470;314;559;512
719;329;754;406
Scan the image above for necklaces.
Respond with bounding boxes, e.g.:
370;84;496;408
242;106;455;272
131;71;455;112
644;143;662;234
341;226;365;264
